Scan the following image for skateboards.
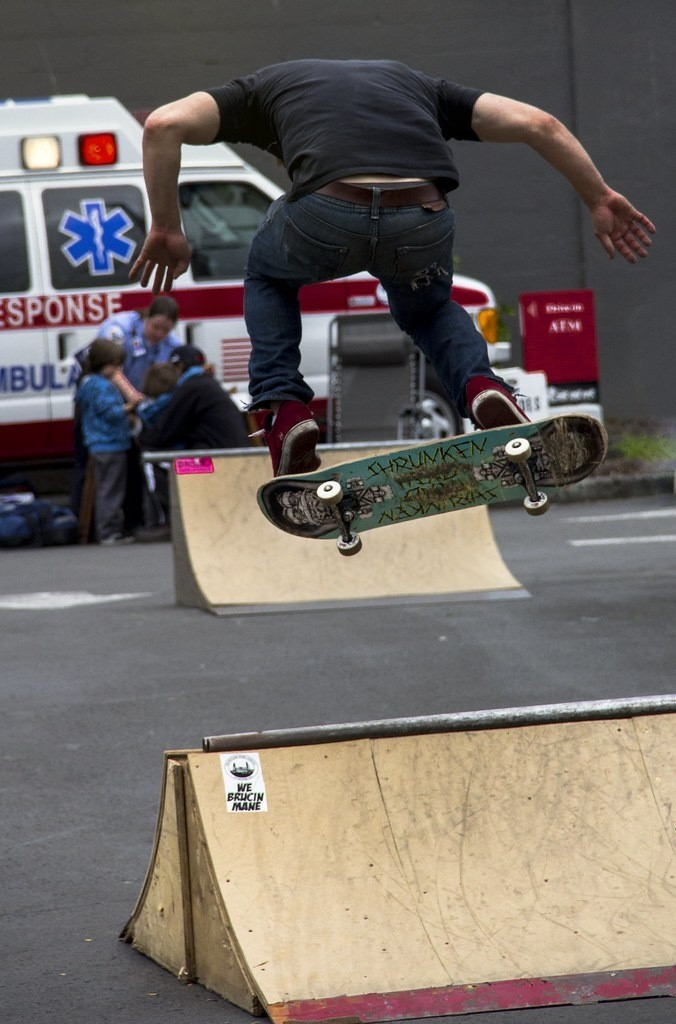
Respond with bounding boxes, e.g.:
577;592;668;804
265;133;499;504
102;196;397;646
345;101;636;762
257;415;607;557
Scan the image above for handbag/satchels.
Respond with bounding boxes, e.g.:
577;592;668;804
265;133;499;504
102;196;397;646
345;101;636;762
0;497;77;551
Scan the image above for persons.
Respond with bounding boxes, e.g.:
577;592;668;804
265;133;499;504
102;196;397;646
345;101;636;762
68;297;249;547
127;58;656;479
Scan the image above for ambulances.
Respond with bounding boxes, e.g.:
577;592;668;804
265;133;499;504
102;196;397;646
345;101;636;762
0;93;549;498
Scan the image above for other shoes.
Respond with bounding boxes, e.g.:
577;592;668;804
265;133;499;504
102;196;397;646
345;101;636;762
135;523;172;542
99;532;136;546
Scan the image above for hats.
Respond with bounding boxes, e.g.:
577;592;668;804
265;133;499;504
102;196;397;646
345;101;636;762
169;346;205;368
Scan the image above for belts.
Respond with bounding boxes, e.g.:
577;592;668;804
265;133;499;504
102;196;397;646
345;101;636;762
314;180;445;209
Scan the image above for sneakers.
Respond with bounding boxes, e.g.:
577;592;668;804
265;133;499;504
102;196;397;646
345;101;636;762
466;376;531;431
248;399;321;477
